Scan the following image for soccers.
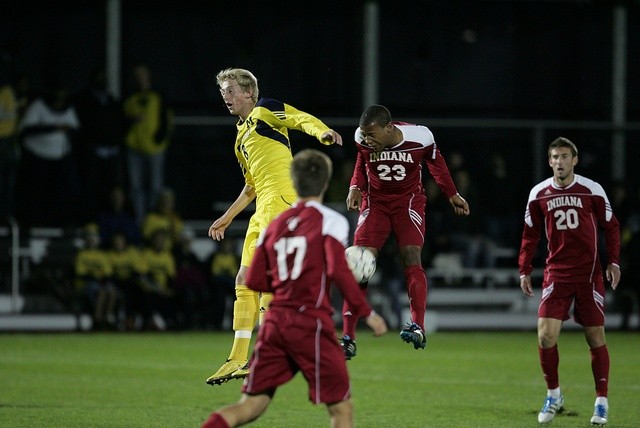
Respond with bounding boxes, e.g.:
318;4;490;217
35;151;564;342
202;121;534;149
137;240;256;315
343;246;376;285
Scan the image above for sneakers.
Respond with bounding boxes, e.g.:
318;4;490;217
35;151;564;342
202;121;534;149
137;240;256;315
207;360;248;386
336;335;357;360
232;361;250;379
537;395;564;424
590;403;608;427
399;317;426;350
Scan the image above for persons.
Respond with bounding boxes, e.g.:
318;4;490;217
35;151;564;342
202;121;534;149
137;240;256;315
74;225;117;326
517;137;622;426
337;105;469;360
197;149;387;428
2;78;22;196
205;67;346;386
71;71;124;224
105;233;139;329
210;235;241;301
116;65;174;225
144;195;185;270
18;71;79;225
136;228;181;328
